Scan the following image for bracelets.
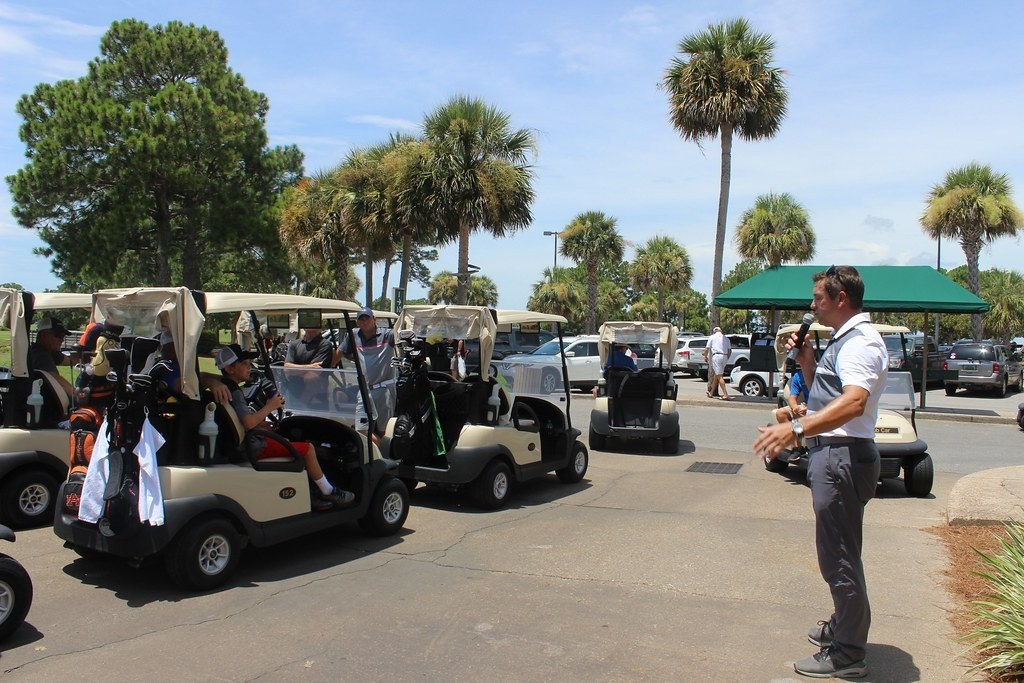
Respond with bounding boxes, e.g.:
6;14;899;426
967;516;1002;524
458;350;461;352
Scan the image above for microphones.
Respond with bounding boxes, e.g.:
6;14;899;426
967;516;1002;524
784;313;814;366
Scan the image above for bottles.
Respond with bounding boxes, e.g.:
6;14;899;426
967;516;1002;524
261;377;277;399
74;366;93;387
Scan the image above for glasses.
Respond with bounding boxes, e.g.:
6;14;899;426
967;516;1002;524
825;264;850;295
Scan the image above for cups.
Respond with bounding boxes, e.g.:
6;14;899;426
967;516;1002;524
598;378;606;397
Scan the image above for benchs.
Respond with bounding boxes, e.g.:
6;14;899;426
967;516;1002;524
603;366;670;398
165;391;305;472
427;371;540;433
0;367;70;426
784;378;805;405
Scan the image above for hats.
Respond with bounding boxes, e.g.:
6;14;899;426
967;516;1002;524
160;329;174;347
36;317;73;336
614;343;628;347
215;344;260;370
713;327;721;333
357;307;374;319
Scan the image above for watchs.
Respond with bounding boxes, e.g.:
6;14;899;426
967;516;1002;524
792;419;804;438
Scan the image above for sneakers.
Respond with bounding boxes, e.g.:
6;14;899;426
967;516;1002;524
794;648;868;678
317;487;355;507
807;620;834;647
787;446;808;461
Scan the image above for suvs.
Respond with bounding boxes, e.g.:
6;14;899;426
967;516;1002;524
499;331;639;395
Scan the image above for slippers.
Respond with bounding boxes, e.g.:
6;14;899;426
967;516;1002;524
719;396;729;400
706;391;712;398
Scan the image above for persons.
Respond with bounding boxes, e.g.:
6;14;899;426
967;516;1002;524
753;265;889;678
30;317;80;396
601;343;638;371
704;327;731;401
447;338;464;379
776;370;809;461
159;330;232;405
331;307;399;446
283;329;333;411
215;343;355;504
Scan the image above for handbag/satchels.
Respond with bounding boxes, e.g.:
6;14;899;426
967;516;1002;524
458;355;466;379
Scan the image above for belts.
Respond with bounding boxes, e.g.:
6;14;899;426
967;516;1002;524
712;352;728;356
369;378;395;389
805;436;873;447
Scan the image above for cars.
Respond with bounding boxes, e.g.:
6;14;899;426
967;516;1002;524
629;328;1024;399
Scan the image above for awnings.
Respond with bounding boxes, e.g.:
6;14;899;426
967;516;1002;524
714;268;989;410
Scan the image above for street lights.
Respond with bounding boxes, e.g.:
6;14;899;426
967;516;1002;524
542;231;558;280
